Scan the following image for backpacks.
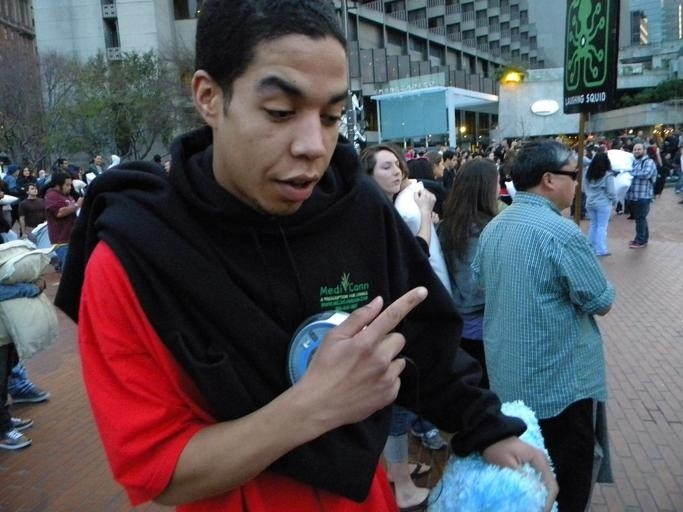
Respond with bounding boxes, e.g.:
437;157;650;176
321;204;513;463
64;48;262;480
640;157;665;197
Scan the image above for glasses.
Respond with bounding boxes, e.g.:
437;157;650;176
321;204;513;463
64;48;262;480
541;167;580;181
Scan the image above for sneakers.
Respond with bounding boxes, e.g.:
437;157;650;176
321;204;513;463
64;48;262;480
0;428;32;450
11;384;50;404
629;241;647;249
411;427;448;450
11;417;34;430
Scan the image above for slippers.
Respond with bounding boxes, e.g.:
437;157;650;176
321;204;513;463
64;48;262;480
396;488;433;511
390;460;431;479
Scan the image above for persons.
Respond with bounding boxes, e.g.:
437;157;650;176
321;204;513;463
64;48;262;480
55;2;558;512
0;150;170;450
365;125;682;512
